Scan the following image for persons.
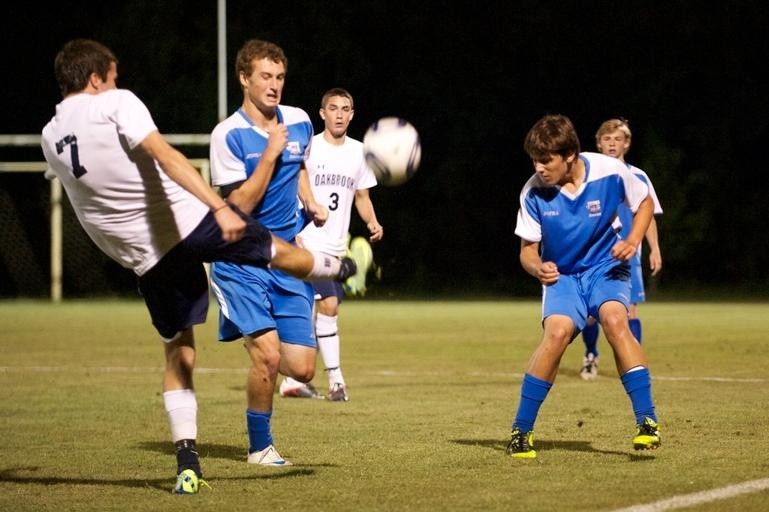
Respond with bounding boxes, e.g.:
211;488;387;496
580;119;663;381
42;39;373;495
280;89;382;400
507;116;662;457
210;41;329;468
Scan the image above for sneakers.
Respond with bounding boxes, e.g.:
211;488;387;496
632;417;662;450
505;429;537;459
171;467;210;495
246;442;294;467
579;353;598;381
327;379;348;403
340;235;374;300
280;373;320;401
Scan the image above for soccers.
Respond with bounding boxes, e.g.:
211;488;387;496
363;118;422;186
